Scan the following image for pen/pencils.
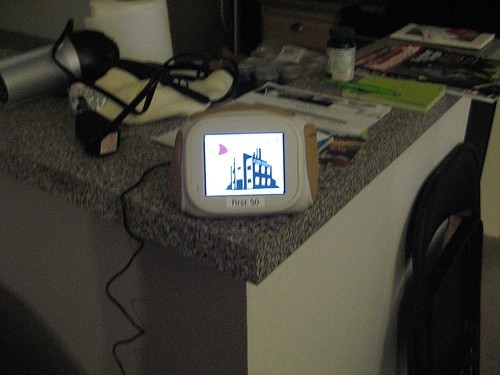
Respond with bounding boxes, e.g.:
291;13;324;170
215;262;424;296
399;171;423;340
330;78;396;98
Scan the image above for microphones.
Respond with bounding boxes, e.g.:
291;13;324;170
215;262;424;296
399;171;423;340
0;31;119;104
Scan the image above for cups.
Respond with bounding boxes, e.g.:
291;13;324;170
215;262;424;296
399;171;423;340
252;51;278;92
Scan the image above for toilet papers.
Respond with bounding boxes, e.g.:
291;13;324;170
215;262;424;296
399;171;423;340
83;0;175;69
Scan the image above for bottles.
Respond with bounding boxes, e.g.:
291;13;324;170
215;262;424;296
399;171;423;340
328;26;355;83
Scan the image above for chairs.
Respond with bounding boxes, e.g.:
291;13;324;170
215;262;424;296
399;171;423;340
406;141;484;375
